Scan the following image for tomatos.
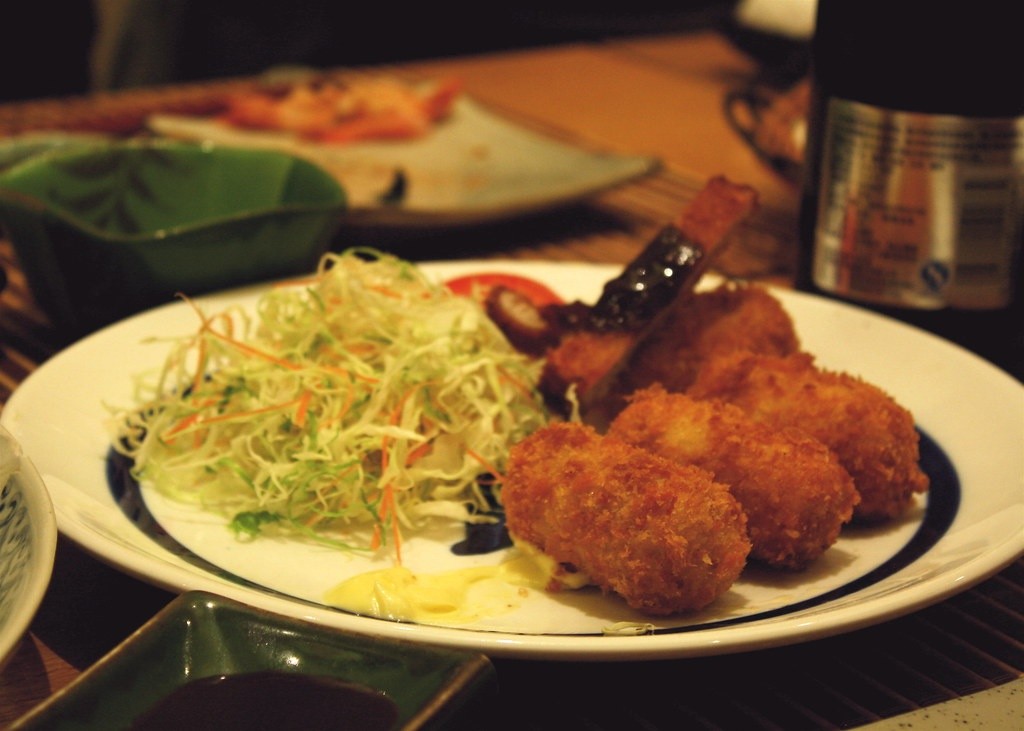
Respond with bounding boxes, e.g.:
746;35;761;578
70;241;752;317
440;272;563;310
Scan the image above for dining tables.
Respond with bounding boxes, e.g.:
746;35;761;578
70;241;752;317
0;35;1023;731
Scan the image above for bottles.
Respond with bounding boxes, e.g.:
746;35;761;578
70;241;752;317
795;0;1024;363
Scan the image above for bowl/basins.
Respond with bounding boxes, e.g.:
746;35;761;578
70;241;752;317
2;144;347;340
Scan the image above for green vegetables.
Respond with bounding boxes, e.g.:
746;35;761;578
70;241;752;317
110;247;583;565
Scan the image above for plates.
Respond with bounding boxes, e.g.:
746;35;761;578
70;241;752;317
0;421;58;675
4;258;1024;668
144;96;656;239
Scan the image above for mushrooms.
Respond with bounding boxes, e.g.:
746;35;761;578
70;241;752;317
485;286;553;353
